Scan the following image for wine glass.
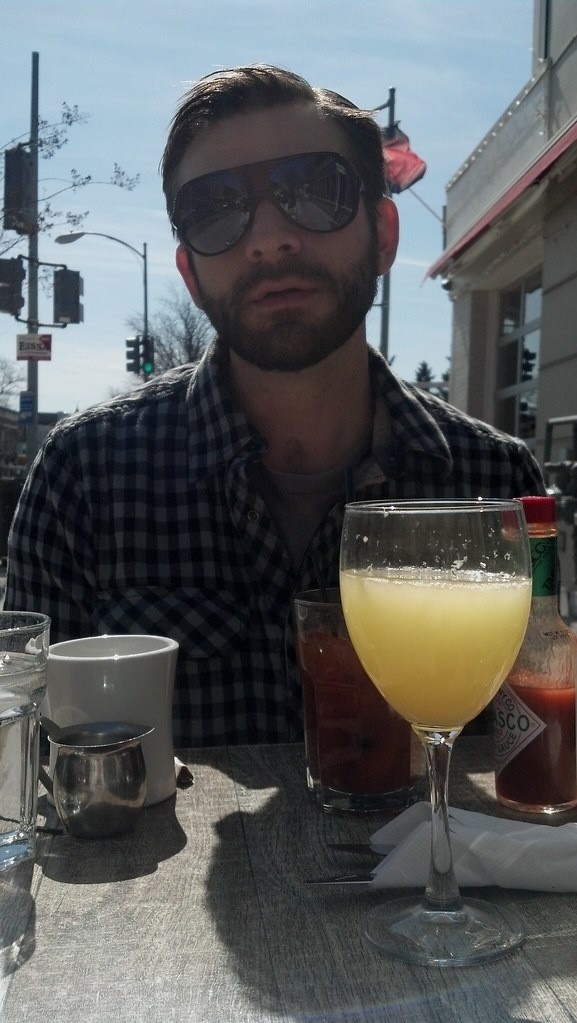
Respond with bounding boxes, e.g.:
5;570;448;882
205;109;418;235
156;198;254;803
339;501;532;968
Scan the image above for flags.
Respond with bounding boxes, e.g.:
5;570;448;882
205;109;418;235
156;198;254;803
379;117;428;194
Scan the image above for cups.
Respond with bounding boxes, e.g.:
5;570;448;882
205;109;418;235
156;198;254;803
36;716;155;838
294;588;413;817
45;635;180;807
0;611;51;873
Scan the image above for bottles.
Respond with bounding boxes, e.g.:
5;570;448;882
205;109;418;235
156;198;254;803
494;496;577;824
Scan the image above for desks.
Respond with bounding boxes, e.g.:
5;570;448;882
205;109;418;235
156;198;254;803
0;746;577;1023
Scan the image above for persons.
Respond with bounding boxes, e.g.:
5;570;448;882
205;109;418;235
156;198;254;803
2;61;567;745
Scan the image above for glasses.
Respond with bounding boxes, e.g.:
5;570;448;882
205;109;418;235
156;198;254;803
170;152;366;257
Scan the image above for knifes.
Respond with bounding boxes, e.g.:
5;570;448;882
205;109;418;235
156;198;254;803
325;844;401;854
303;872;378;884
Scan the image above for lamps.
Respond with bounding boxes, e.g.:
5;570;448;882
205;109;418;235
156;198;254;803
442;279;452;290
